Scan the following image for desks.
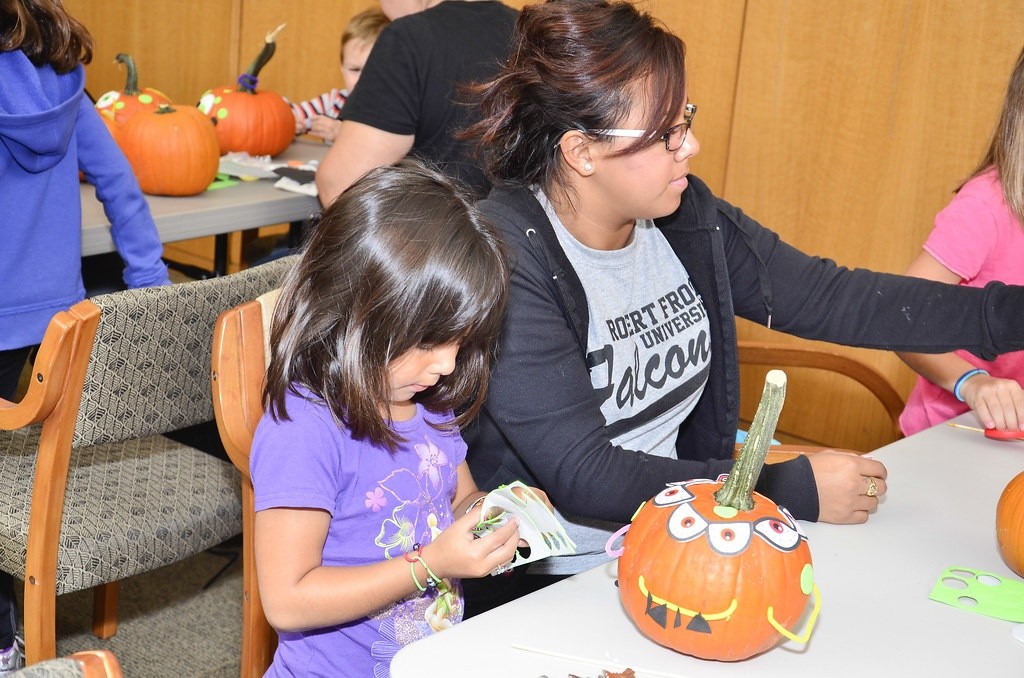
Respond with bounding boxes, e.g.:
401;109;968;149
388;408;1024;678
81;139;332;284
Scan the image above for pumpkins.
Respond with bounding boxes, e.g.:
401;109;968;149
619;368;813;662
94;37;296;197
995;469;1024;578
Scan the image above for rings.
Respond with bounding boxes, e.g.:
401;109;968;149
865;477;878;497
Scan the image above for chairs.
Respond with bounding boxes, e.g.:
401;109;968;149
0;250;904;677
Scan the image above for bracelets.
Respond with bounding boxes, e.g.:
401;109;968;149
954;367;990;401
465;497;486;516
402;544;444;593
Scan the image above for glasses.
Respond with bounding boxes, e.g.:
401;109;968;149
554;103;698;153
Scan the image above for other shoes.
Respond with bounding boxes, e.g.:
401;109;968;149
0;636;25;674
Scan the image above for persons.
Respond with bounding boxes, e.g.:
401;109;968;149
278;5;394;146
0;0;175;678
454;0;1024;623
315;0;531;217
887;44;1024;443
249;160;553;678
